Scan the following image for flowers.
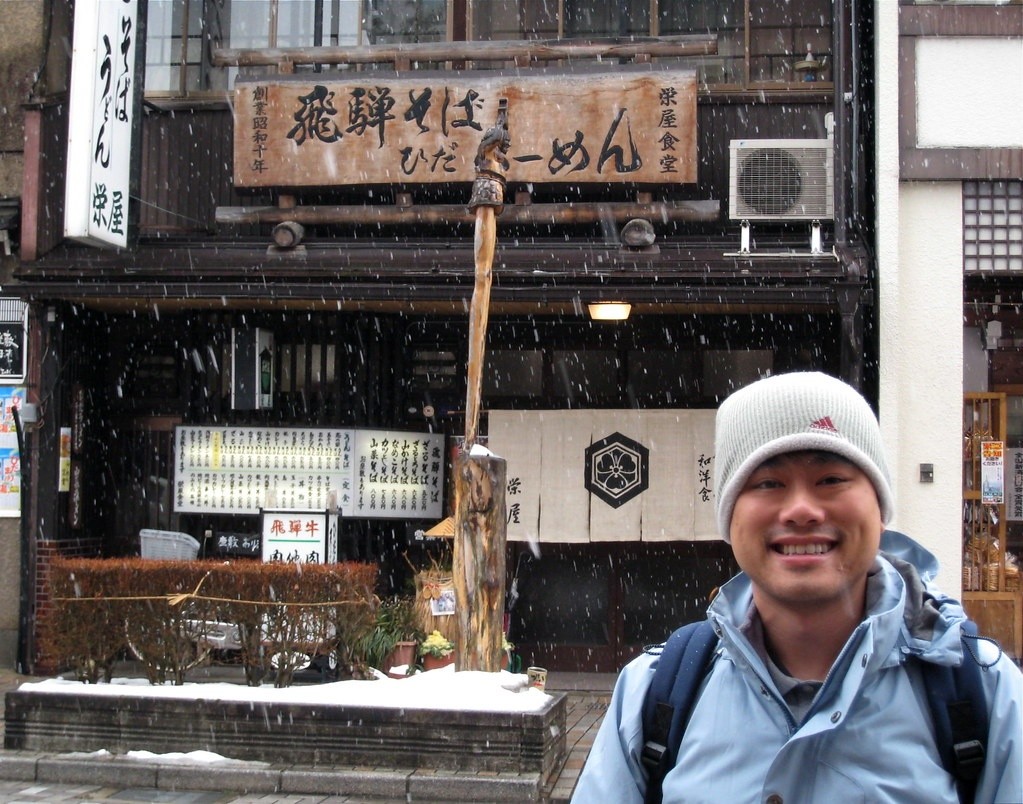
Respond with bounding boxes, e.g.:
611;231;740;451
418;629;455;660
501;632;515;650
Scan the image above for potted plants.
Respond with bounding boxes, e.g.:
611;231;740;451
349;593;425;679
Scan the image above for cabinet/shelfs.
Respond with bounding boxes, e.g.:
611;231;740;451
962;391;1023;665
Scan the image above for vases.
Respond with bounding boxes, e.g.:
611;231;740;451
501;651;509;670
421;652;456;672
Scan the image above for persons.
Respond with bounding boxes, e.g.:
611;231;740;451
570;371;1023;804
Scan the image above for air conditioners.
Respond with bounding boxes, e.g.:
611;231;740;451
727;138;835;220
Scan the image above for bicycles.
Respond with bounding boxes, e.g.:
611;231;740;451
122;585;339;674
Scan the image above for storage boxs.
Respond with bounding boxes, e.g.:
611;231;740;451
139;529;200;561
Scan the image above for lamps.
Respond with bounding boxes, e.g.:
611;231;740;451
620;219;656;247
585;301;635;321
271;221;304;247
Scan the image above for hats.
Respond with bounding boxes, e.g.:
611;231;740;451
713;371;893;545
